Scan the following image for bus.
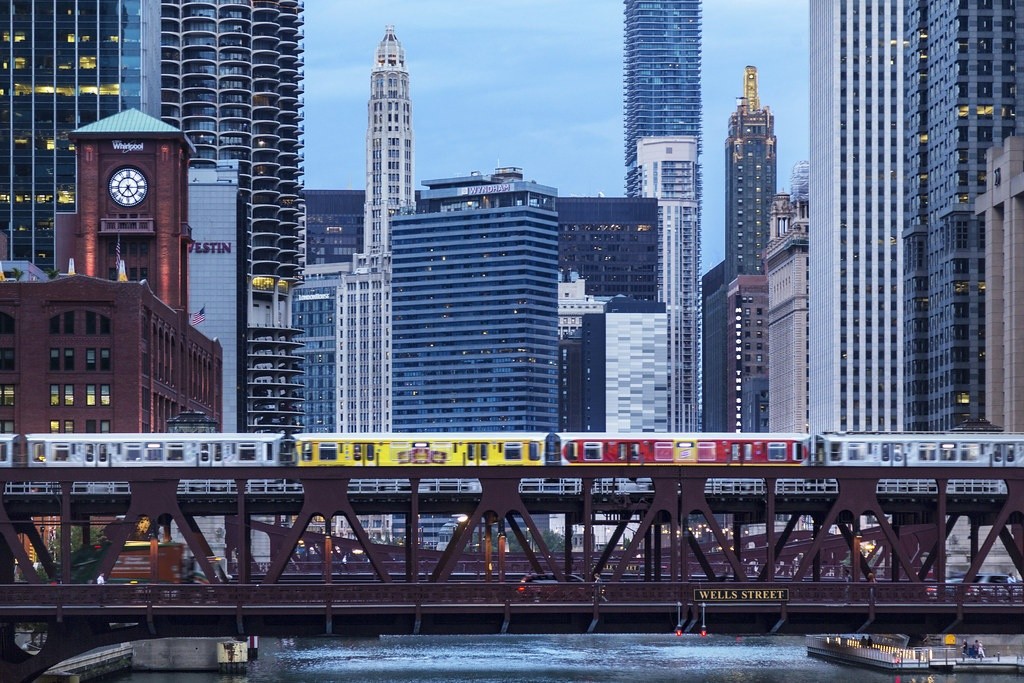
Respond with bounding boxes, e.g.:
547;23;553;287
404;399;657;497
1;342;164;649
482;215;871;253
103;541;184;598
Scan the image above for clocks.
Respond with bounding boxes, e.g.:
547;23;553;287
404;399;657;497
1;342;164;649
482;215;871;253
106;165;150;209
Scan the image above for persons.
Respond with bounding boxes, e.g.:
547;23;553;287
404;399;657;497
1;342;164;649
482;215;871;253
858;635;873;648
865;568;876;584
1006;572;1016;584
96;572;105;584
593;573;609;602
959;639;985;658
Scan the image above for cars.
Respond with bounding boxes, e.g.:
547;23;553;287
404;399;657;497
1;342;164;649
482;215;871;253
516;572;602;604
966;573;1024;601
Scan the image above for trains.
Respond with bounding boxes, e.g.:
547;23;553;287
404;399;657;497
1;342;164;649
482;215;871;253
0;429;1024;500
